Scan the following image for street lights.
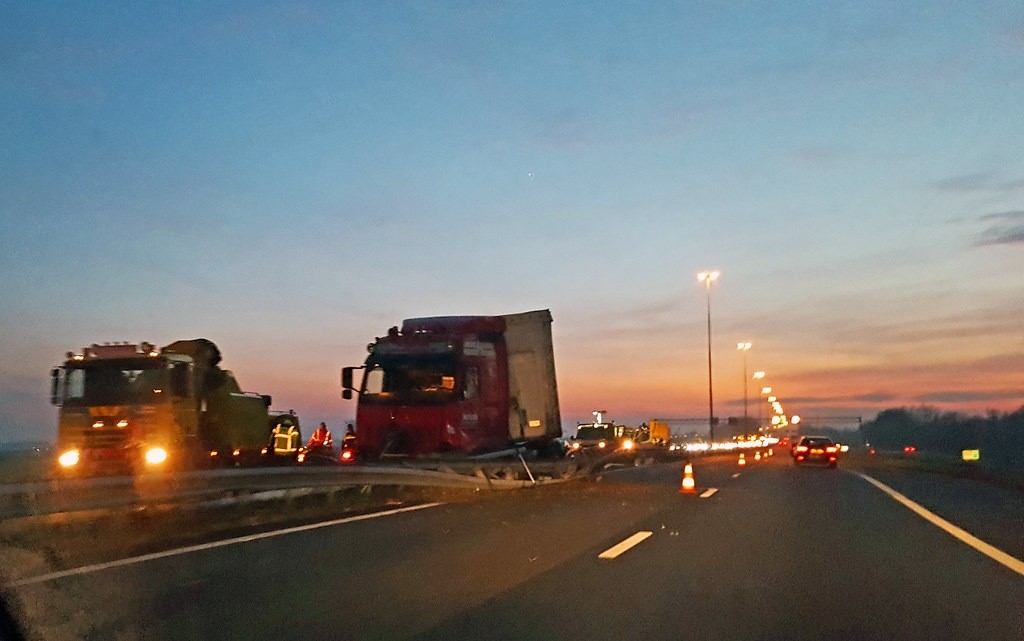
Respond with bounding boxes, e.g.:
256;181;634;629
738;342;751;441
696;271;719;443
758;387;786;428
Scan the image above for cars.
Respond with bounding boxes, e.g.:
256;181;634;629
779;435;839;468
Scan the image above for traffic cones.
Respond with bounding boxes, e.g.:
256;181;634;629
680;463;697;494
738;453;746;470
754;449;774;462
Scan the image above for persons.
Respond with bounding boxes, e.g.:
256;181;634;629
267;416;302;467
308;422;333;448
341;424;356;451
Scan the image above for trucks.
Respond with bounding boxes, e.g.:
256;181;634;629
52;339;300;476
570;420;669;451
341;310;565;463
